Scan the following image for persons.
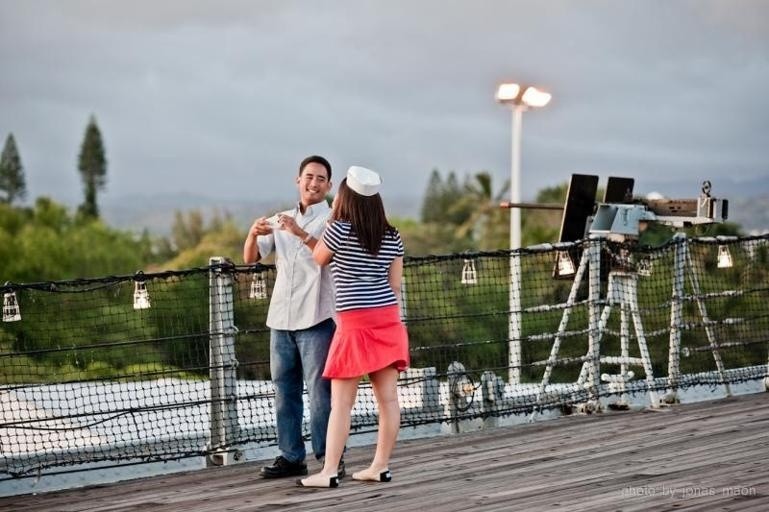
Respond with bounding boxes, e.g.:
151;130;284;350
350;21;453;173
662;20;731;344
243;155;345;481
296;166;410;488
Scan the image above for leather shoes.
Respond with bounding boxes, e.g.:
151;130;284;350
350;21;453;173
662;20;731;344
259;456;308;478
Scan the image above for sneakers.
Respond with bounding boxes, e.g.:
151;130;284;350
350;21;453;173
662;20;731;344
295;475;339;488
352;466;392;482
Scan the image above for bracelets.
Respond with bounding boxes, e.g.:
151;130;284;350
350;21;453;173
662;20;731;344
303;234;313;244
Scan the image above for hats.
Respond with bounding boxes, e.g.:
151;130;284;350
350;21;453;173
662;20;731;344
346;166;384;197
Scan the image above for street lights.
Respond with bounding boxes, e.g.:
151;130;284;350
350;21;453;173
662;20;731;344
495;78;552;386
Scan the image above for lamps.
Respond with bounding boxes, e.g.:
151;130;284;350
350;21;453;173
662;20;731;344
460;258;477;285
133;279;151;310
558;249;576;277
3;291;22;322
717;244;733;269
249;271;268;300
